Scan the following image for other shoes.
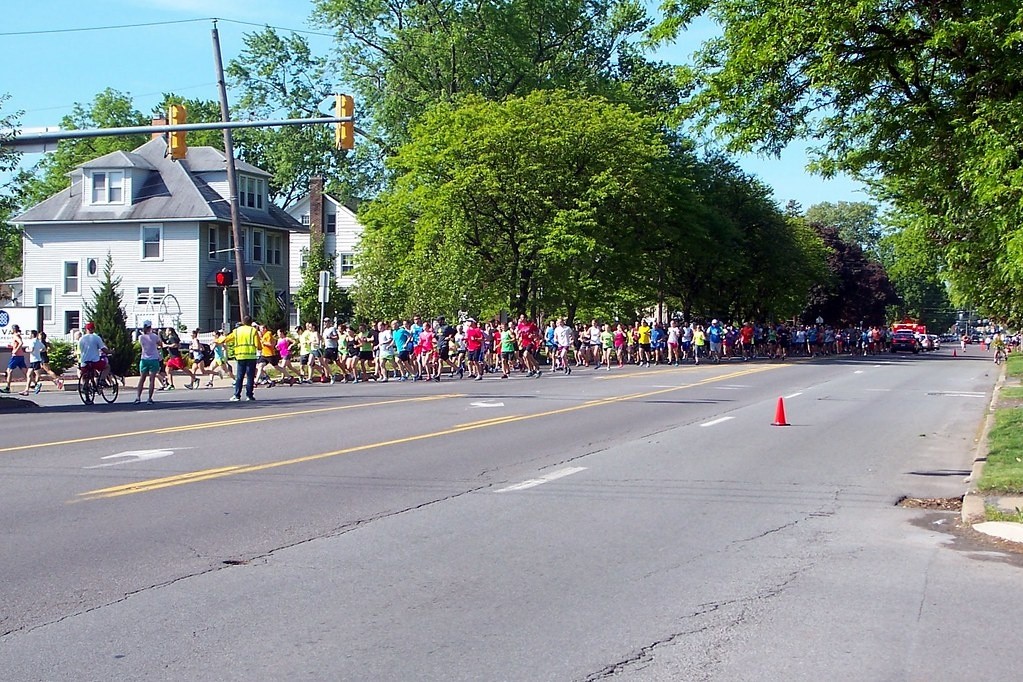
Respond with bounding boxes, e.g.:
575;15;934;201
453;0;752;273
86;401;94;405
712;352;788;366
31;386;35;388
57;378;64;390
618;356;699;368
100;380;109;388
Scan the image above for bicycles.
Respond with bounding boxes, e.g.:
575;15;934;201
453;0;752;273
77;354;119;405
994;345;1004;365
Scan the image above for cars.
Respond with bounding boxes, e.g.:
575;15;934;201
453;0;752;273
888;317;1011;354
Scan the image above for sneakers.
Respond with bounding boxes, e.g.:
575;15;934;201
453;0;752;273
253;369;510;388
519;357;612;379
230;397;240;402
985;349;990;352
34;383;42;394
121;377;126;386
147;401;154;404
1005;357;1007;361
0;389;11;393
19;391;29;396
246;398;255;400
158;370;237;391
133;400;140;405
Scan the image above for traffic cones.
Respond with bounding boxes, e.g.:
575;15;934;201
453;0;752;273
951;348;958;357
770;395;790;426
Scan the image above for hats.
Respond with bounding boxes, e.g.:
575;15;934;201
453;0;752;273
144;320;152;326
712;319;717;324
12;325;19;329
86;322;94;328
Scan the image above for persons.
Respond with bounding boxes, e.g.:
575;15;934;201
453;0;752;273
34;332;64;389
133;313;1023;405
0;325;42;394
18;330;46;396
67;322;126;406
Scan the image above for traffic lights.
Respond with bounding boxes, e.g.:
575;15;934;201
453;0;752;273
215;271;234;287
